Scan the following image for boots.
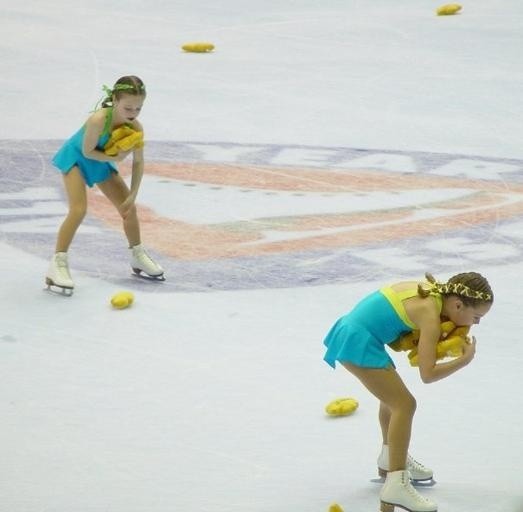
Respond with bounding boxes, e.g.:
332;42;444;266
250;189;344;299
46;252;75;289
380;470;437;511
376;444;434;481
128;244;164;277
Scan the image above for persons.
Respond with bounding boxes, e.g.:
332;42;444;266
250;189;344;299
318;269;496;512
40;75;163;289
